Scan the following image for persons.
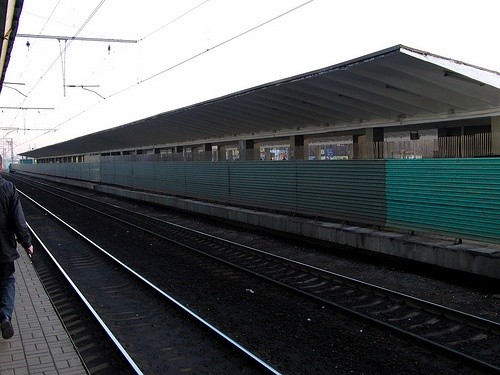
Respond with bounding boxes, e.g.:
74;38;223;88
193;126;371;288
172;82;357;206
0;155;33;339
259;156;331;160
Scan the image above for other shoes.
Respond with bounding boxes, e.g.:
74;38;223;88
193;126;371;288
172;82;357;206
2;316;14;339
